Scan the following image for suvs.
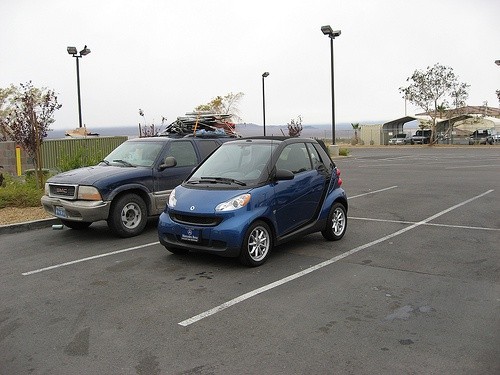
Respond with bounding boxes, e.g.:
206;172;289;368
411;129;432;145
39;131;242;239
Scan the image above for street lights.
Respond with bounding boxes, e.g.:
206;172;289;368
320;25;341;146
261;71;270;136
67;45;91;127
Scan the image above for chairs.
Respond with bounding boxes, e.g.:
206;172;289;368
287;147;306;172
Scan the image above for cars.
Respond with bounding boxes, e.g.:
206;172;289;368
469;129;493;146
388;134;411;145
158;134;349;269
